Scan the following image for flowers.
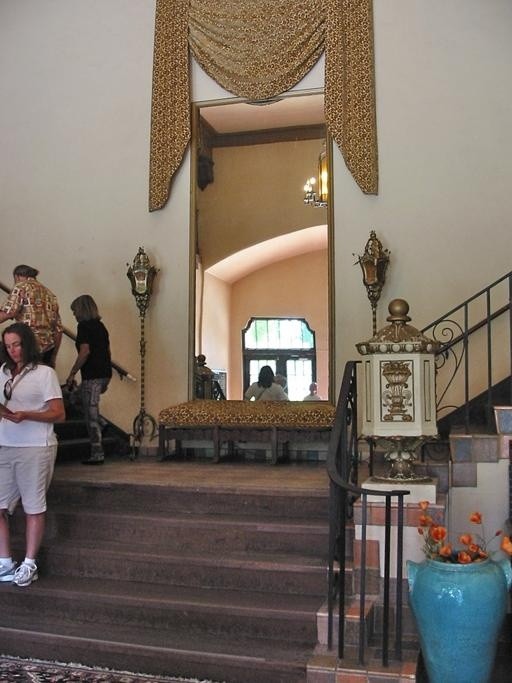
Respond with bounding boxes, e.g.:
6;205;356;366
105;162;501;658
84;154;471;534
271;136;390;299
412;498;511;565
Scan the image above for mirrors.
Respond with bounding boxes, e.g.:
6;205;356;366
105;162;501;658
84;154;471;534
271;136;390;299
193;92;329;404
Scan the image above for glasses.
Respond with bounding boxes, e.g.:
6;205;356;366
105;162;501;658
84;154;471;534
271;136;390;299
4;379;13;400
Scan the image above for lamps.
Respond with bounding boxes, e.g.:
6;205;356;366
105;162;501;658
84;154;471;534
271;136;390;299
300;140;329;209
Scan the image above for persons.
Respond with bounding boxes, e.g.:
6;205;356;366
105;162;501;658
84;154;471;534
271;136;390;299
0;322;65;587
303;380;325;401
0;263;64;373
62;294;113;465
244;364;291;402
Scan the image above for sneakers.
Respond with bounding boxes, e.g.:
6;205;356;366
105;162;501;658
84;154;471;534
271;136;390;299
0;560;18;582
82;460;104;465
11;561;39;588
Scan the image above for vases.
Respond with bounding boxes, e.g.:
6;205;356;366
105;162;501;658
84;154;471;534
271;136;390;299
408;559;511;683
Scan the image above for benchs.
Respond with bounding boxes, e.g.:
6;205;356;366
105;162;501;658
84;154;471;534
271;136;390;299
156;399;340;468
